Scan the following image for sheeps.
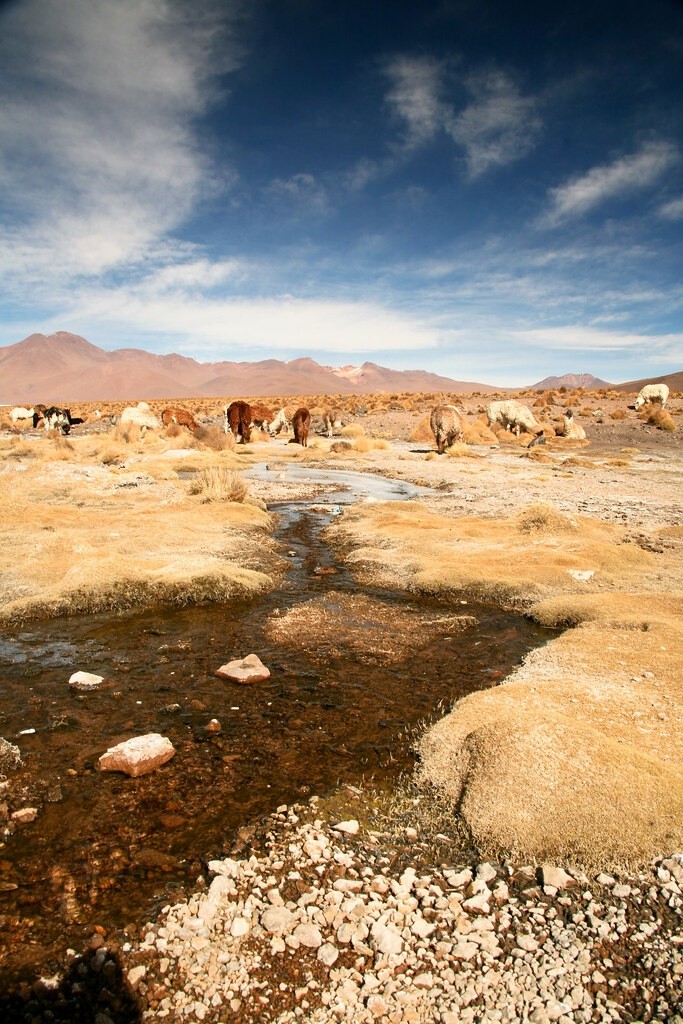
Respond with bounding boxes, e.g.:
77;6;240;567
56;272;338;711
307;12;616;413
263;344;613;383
562;413;587;440
223;402;274;444
635;383;669;410
120;402;160;431
9;404;82;435
269;405;311;448
322;410;339;438
161;409;199;432
486;402;542;437
429;405;463;453
